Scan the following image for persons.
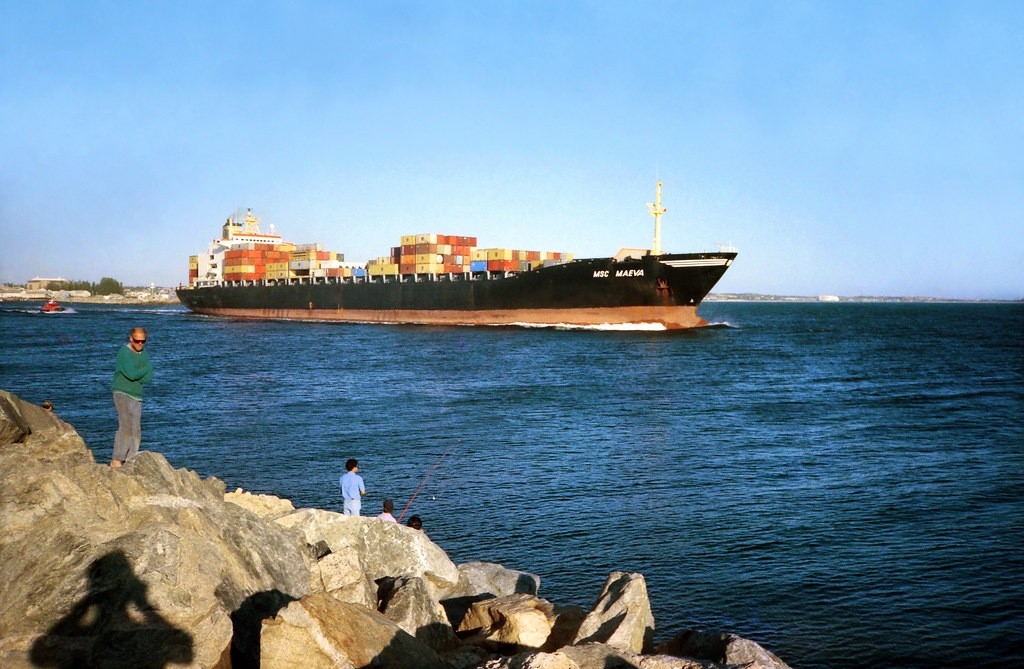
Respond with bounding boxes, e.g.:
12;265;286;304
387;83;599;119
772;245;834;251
108;326;155;468
338;458;366;516
406;514;424;533
376;498;397;524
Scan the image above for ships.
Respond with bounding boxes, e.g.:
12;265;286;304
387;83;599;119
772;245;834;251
173;197;743;332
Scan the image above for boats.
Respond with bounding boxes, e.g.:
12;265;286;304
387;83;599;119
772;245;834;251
40;294;65;314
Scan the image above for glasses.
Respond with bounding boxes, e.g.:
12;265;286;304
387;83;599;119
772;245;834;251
131;335;146;344
